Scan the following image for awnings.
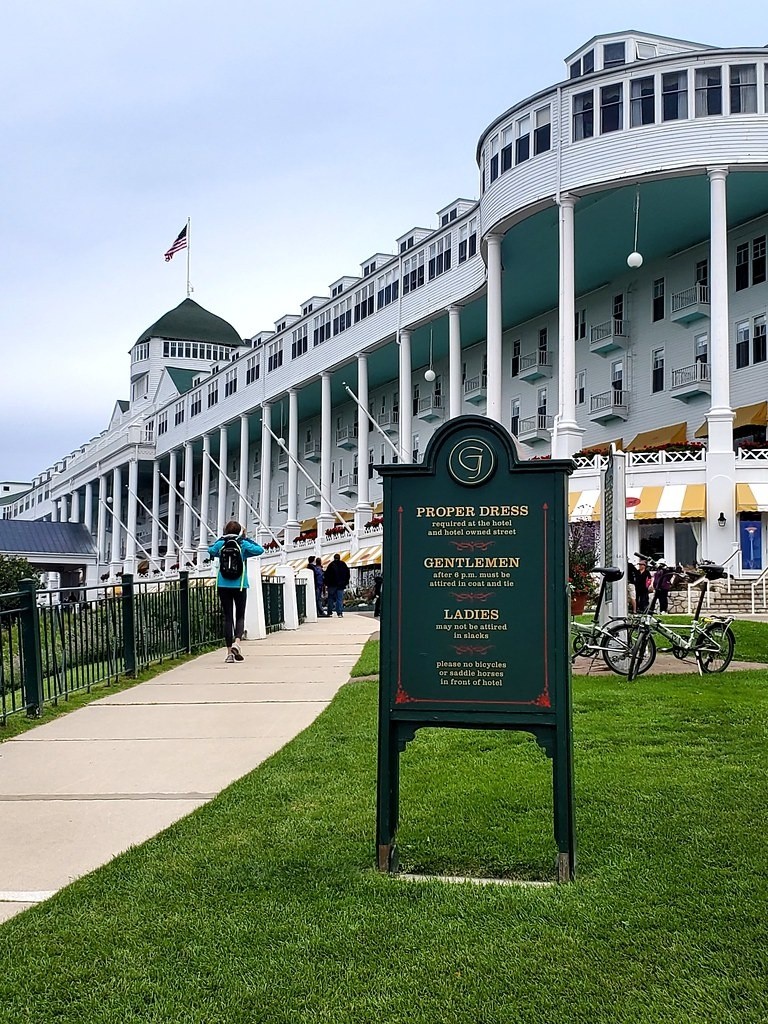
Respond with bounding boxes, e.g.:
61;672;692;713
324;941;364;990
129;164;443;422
567;483;706;522
735;483;768;513
300;501;385;534
260;544;381;577
582;401;768;452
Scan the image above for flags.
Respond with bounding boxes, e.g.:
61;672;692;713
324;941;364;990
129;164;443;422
164;219;190;262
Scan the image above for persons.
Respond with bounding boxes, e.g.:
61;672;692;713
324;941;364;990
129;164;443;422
307;554;350;618
626;558;668;615
208;521;262;664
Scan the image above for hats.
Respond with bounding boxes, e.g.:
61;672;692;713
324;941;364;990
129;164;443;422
637;561;646;566
655;558;668;566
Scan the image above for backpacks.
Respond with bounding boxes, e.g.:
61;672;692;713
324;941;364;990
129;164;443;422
219;537;243;581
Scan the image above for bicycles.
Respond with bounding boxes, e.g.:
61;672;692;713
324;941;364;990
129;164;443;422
604;550;736;677
573;567;660;677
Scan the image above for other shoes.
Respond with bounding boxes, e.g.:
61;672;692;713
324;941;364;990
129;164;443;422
319;613;327;616
327;613;332;616
338;613;343;618
660;610;668;615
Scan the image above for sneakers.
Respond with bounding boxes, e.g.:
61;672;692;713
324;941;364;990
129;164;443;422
231;643;244;661
225;654;234;663
